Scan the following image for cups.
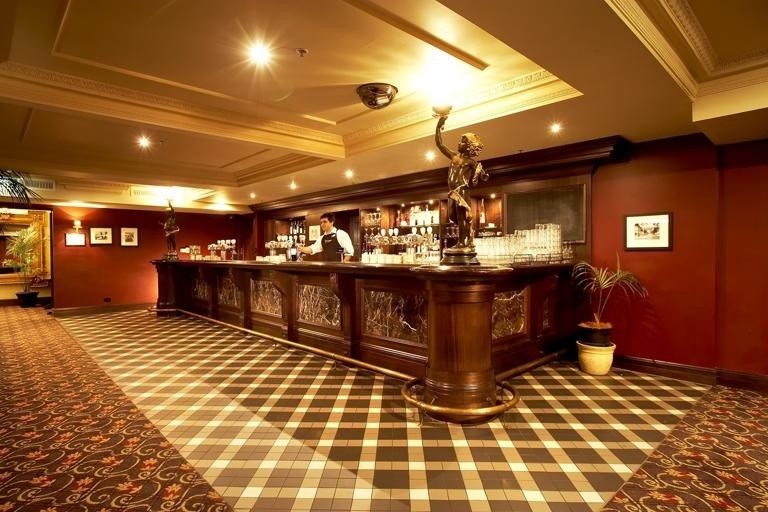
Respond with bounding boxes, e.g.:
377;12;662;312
377;235;434;246
362;250;440;264
474;223;562;264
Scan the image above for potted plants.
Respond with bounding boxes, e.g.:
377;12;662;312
572;255;650;375
2;222;49;307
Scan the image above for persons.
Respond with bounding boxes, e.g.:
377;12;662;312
158;199;178;253
435;116;490;248
297;212;355;262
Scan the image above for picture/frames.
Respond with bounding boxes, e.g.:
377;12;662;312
120;227;138;247
625;212;673;252
89;228;114;245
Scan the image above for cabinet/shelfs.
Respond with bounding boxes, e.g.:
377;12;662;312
274;216;306;261
360;199;441;263
470;194;502;237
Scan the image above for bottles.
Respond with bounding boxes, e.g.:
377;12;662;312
290;221;304;234
361;243;374;254
395;204;431;225
441;238;450;258
289;238;298;262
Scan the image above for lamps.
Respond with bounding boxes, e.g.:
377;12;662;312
72;219;83;232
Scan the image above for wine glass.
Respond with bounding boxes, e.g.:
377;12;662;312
445;227;459;237
363;211;382;238
